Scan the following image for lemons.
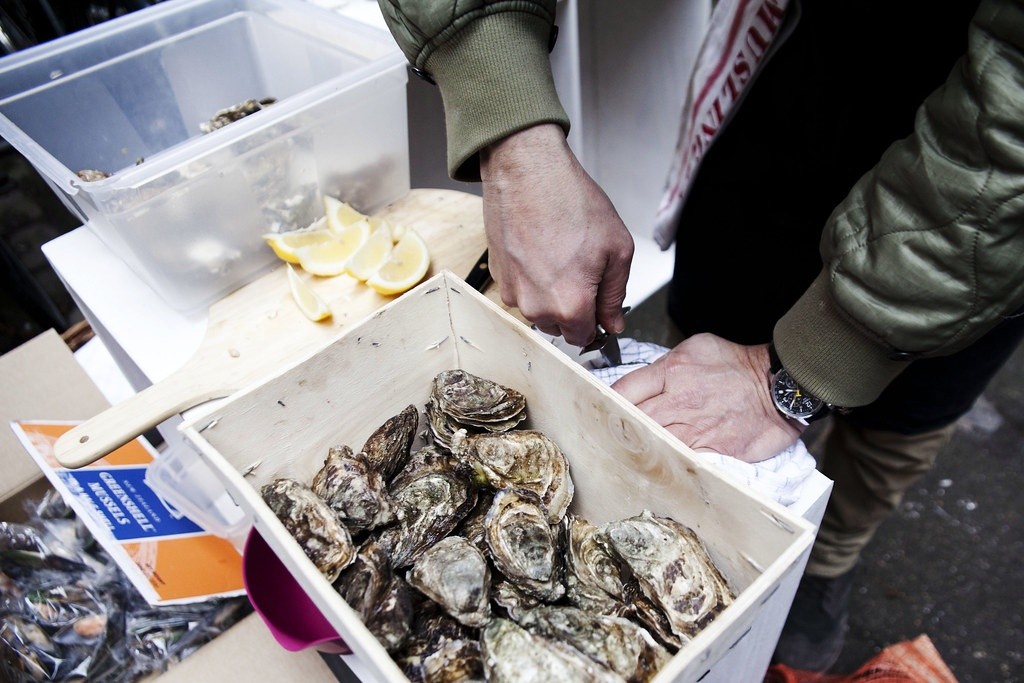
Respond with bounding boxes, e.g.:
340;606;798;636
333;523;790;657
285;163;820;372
260;195;430;321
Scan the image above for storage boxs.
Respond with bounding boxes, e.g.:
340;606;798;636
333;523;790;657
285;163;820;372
0;0;408;324
0;328;344;683
174;266;819;683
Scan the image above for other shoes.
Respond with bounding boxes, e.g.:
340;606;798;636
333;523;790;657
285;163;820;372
771;571;851;673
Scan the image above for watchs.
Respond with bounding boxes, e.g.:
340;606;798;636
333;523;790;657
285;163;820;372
767;341;834;424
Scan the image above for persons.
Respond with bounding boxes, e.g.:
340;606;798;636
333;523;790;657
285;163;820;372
378;0;1024;671
